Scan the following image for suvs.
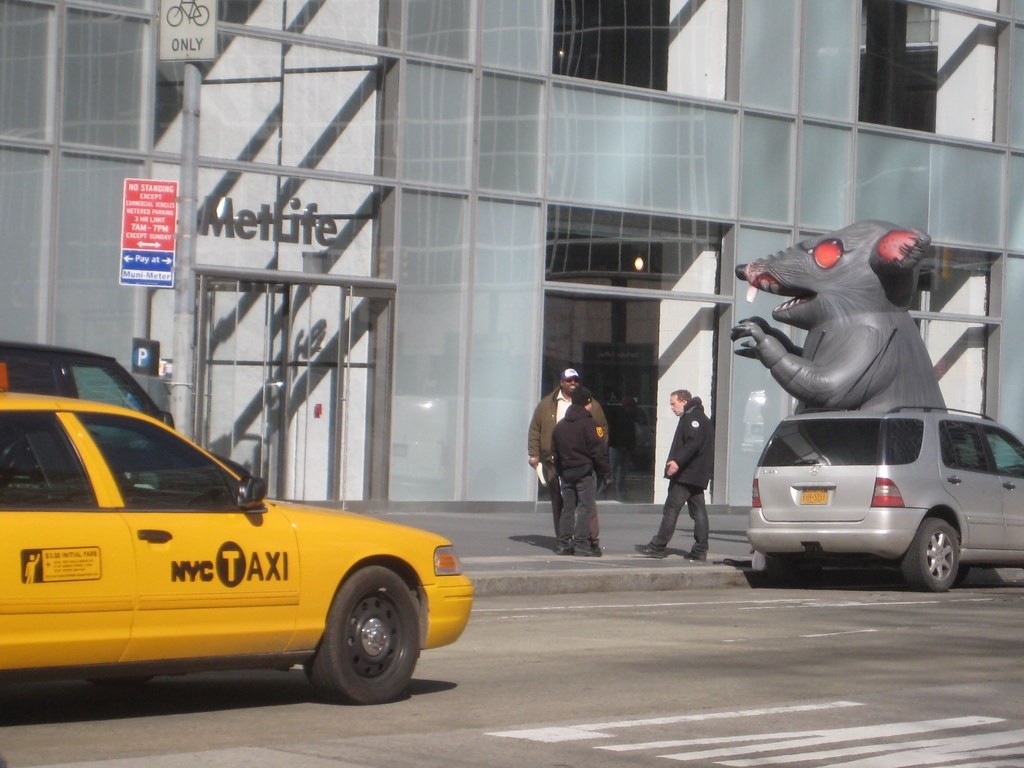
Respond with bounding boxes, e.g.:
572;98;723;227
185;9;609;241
749;406;1024;591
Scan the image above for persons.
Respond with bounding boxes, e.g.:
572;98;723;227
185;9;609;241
550;384;609;557
633;389;715;563
527;368;609;558
595;393;648;503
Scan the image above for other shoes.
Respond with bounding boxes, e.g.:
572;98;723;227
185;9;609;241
684;553;706;561
573;544;602;557
556;547;574;555
634;544;668;557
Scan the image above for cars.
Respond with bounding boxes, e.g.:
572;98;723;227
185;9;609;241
0;340;175;431
2;391;474;703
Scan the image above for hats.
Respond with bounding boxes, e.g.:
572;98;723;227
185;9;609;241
561;368;579;380
572;390;592;406
